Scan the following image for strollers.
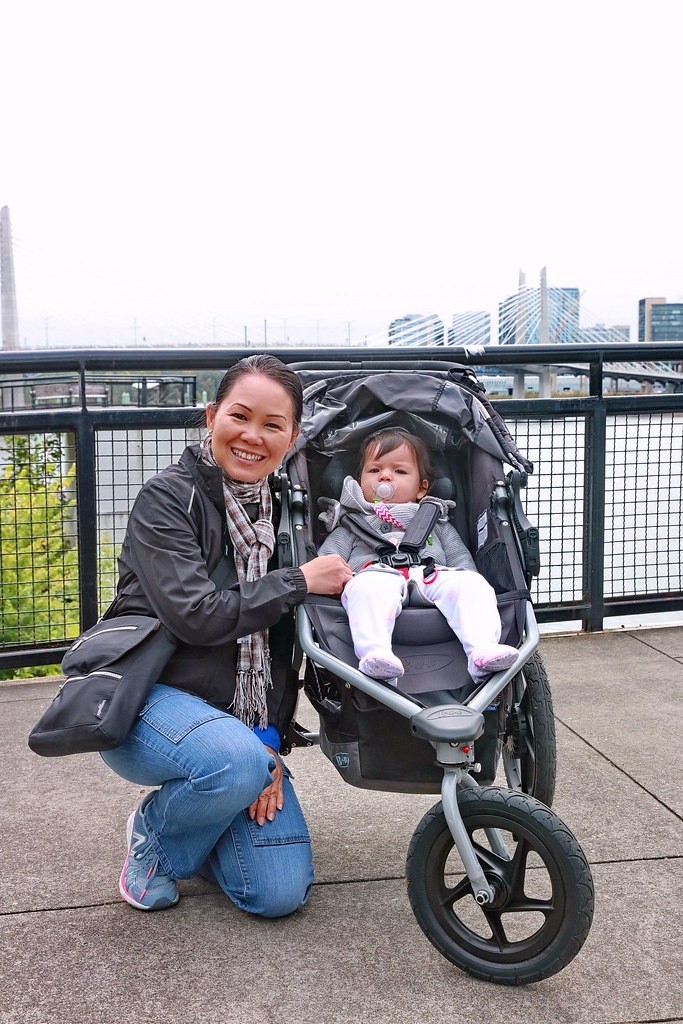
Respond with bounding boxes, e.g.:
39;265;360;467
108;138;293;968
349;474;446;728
258;358;597;987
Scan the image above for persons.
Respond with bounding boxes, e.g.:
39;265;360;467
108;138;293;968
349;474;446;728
97;352;354;914
316;427;517;687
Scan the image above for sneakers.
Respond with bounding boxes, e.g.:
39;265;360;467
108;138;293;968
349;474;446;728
118;789;181;911
197;856;219;883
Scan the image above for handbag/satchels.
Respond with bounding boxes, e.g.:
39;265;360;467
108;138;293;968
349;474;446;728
28;597;175;757
